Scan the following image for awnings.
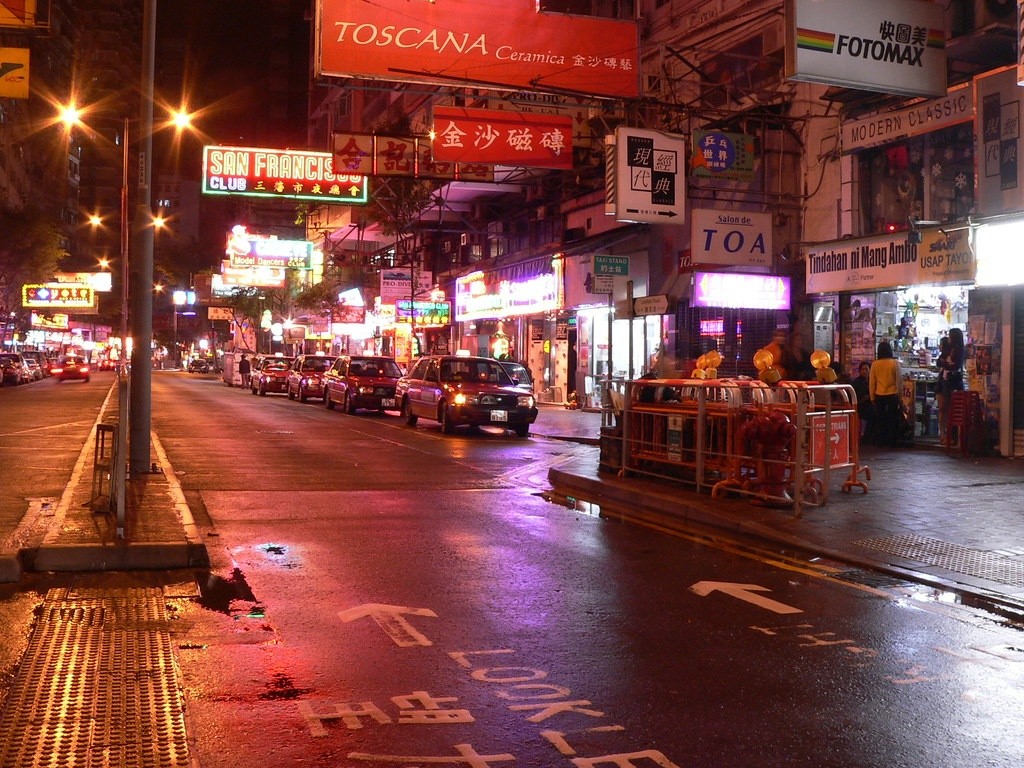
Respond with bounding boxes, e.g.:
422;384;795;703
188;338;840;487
483;220;659;305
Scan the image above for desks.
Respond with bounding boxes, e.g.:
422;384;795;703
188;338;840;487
913;380;940;437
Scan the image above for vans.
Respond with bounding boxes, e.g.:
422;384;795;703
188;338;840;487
222;351;254;387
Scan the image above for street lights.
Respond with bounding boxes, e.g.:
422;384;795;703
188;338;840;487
53;101;195;373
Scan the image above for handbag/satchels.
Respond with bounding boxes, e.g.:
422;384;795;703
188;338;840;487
946;372;965;391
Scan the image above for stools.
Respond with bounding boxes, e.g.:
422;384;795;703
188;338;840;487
945;389;984;462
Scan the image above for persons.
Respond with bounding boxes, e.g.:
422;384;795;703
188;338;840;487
239;355;251;389
769;327;965;447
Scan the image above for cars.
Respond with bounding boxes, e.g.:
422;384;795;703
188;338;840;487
284;351;342;403
249;352;298;396
0;350;62;386
319;356;405;415
487;361;535;395
55;355;91;382
189;359;211;374
395;354;538;438
99;360;117;372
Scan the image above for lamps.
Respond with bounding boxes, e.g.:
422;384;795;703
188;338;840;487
705;349;722;379
690;353;708;380
753;349;782;385
904;214;923;244
810;349;838;384
